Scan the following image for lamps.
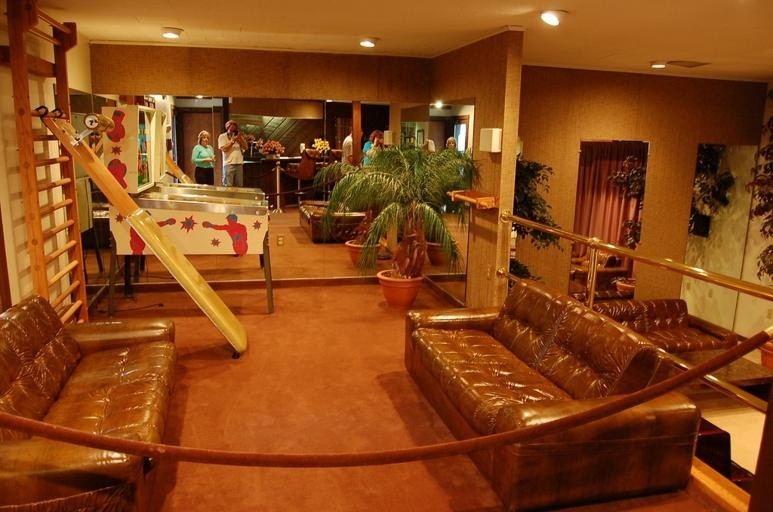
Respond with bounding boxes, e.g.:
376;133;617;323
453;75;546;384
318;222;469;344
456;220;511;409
358;37;376;48
649;58;667;69
160;26;183;39
539;8;567;27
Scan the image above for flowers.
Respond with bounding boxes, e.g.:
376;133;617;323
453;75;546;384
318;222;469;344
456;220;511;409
260;139;285;154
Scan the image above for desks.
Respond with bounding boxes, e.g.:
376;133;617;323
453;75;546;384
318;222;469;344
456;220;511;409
674;348;772;395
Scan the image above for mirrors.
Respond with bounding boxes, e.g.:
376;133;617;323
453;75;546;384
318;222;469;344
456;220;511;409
227;112;325;160
396;96;476;308
68;93;391;293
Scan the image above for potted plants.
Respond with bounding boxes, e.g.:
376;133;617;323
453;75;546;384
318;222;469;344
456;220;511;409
312;141;480;310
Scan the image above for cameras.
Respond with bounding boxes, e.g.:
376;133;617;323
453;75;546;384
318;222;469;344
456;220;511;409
227;129;239;137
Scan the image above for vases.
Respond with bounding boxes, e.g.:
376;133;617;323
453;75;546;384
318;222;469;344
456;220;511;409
264;152;280;160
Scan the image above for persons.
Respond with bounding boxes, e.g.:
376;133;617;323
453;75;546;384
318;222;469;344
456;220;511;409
360;130;384;165
218;119;248;187
191;130;216;186
445;136;459;214
341;125;363;175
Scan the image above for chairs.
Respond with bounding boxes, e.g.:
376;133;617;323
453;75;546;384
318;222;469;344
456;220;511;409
284;148;321;209
569;244;631;288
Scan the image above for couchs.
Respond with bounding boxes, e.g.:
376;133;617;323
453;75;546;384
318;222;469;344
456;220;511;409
403;278;701;511
592;297;737;354
0;294;178;512
297;199;366;244
